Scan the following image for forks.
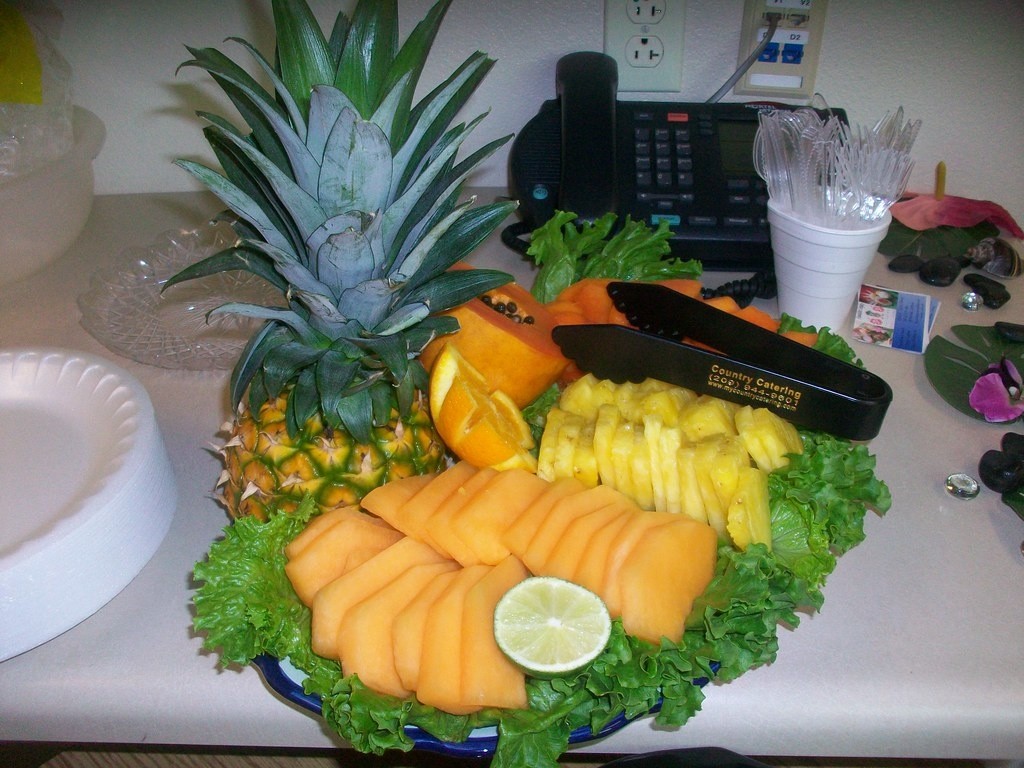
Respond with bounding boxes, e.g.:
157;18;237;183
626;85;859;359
752;90;922;232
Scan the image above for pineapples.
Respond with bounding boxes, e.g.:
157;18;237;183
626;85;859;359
158;0;519;532
538;374;804;556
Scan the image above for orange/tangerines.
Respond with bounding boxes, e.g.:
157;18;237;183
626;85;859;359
426;342;535;465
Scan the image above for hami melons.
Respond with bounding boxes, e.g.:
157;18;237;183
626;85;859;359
283;459;718;716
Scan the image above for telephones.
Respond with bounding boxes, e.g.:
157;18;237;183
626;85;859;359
509;49;859;277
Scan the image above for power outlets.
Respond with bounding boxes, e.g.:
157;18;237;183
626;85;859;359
598;2;687;94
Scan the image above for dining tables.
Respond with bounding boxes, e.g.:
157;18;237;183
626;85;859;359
0;175;1024;767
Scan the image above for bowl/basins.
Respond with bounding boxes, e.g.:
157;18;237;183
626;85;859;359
0;105;107;283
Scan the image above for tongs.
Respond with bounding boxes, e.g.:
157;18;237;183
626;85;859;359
544;282;898;443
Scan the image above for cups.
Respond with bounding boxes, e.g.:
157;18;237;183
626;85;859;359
765;188;891;335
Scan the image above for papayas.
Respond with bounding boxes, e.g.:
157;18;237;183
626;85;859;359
420;263;819;407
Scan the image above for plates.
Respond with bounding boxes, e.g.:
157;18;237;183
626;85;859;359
73;218;286;375
256;650;719;756
0;349;177;666
